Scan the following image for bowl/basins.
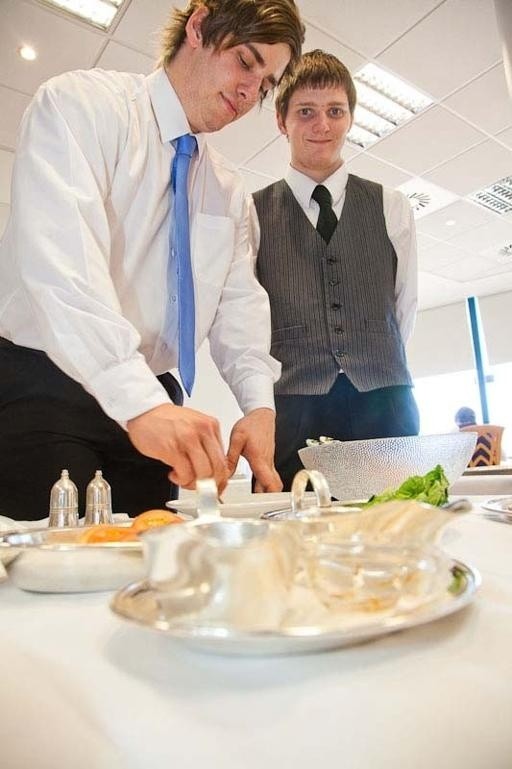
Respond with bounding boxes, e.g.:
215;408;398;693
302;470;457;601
296;433;478;502
0;525;151;595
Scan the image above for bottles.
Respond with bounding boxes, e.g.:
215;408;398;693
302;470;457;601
48;469;113;528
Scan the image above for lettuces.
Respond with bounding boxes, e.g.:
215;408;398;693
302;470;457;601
365;464;449;507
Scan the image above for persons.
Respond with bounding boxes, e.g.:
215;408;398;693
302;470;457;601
251;49;422;492
0;2;304;521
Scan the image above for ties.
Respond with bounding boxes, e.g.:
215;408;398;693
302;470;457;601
172;134;197;398
308;186;339;244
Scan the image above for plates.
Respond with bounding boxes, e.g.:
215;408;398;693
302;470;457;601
165;490;321;518
484;495;512;518
109;555;478;656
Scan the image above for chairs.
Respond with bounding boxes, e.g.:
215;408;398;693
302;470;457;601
459;424;504;468
